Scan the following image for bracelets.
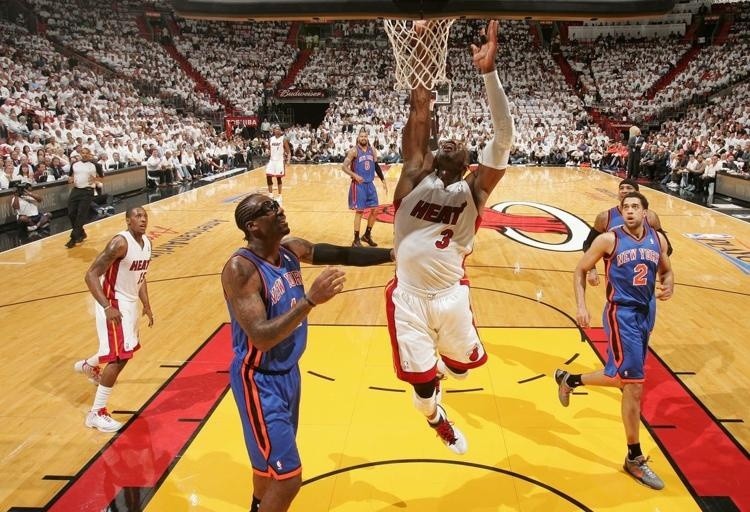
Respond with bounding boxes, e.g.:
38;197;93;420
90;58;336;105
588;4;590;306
103;304;113;310
304;293;317;309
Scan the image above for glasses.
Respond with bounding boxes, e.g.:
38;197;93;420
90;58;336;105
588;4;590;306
252;199;279;220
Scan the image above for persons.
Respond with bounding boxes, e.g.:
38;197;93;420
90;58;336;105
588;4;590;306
0;1;750;193
341;131;387;248
10;182;55;232
219;192;396;511
92;175;116;214
74;206;154;432
554;191;674;490
583;178;674;288
265;125;292;202
384;19;517;455
64;148;103;248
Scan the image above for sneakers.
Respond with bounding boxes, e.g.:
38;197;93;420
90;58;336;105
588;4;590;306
64;231;87;247
623;454;664;490
352;241;362;247
74;359;103;385
555;369;573;407
85;408;122;432
435;370;444;402
362;235;377;246
427;404;466;455
